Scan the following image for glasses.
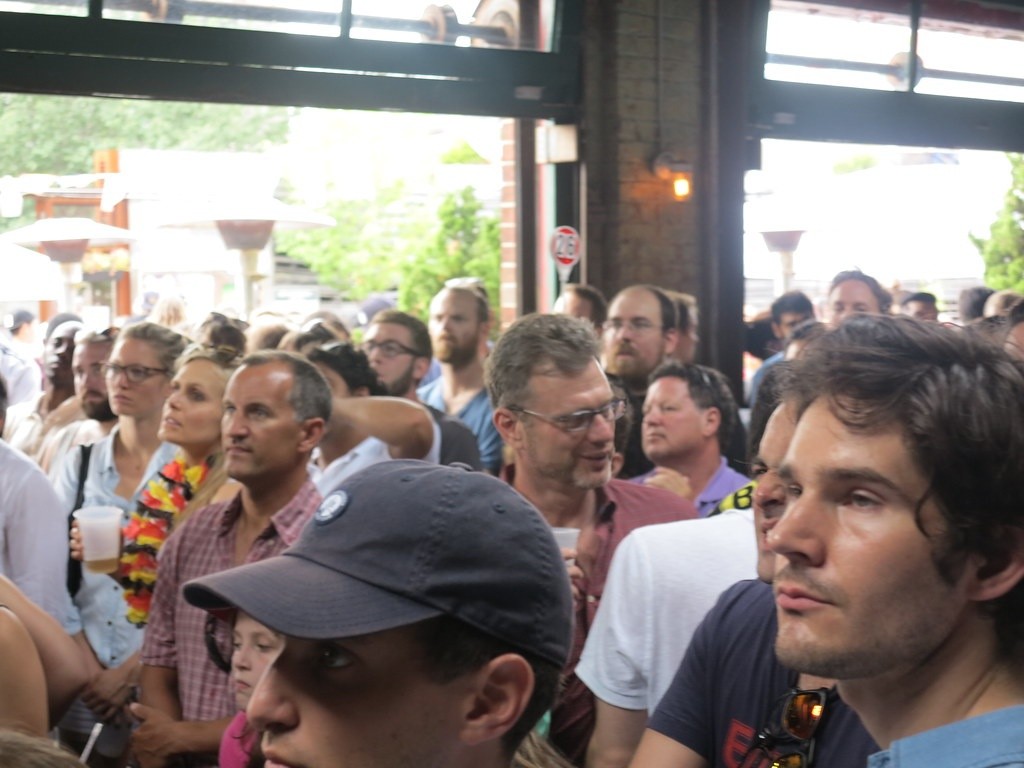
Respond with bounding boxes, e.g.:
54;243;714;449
502;397;628;432
601;318;661;335
197;312;249;331
301;318;334;339
763;686;830;768
359;339;424;358
100;361;169;383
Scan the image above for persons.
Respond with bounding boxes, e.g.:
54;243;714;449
0;270;1024;768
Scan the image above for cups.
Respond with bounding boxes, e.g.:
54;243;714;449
72;506;124;574
552;528;580;564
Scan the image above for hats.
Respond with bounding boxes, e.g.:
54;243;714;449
181;458;576;671
